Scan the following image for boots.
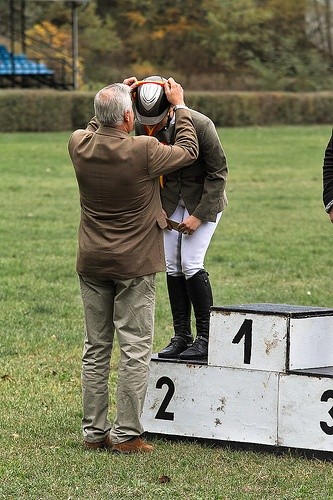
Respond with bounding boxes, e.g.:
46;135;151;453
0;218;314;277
179;268;213;359
158;272;194;359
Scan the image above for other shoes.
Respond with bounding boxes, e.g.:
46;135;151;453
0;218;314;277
114;437;156;452
84;436;113;449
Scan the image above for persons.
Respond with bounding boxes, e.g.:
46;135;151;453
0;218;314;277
135;75;229;359
69;76;199;456
323;128;333;225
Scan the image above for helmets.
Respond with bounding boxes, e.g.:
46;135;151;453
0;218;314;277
131;76;172;125
